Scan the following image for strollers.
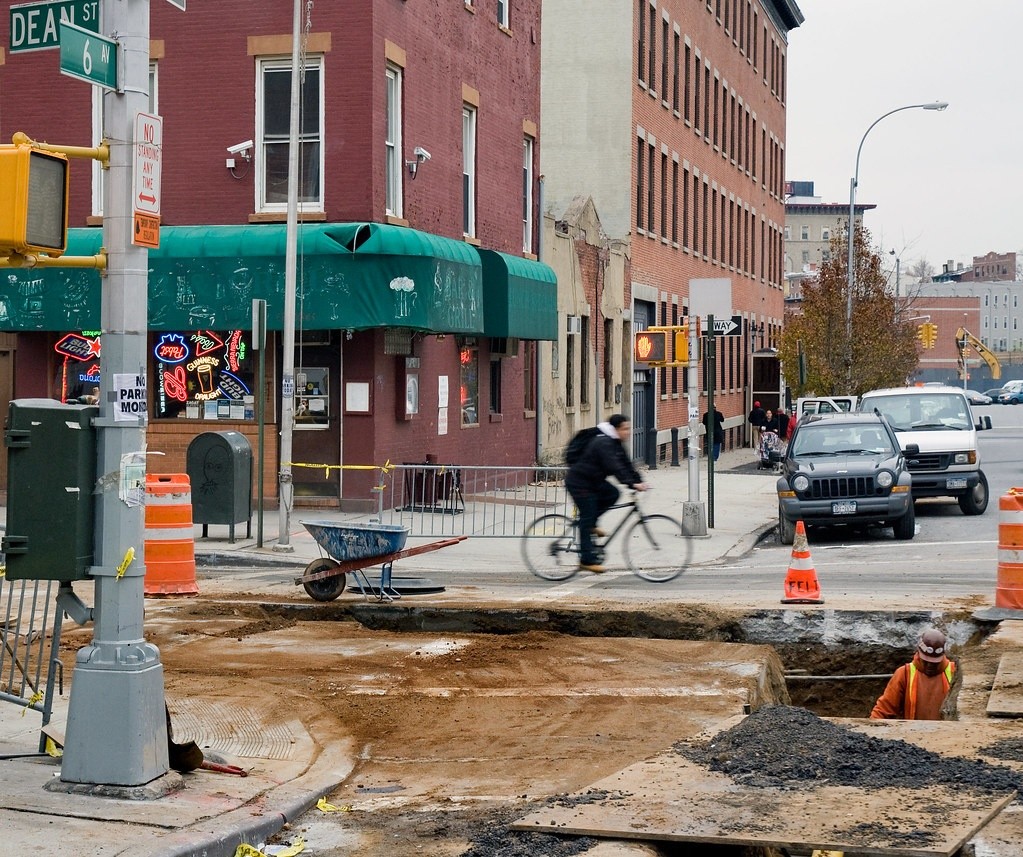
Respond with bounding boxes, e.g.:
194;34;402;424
753;429;782;472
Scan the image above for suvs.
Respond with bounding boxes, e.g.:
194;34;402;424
769;407;919;543
796;384;993;516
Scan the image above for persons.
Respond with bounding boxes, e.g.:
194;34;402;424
748;402;766;451
787;413;797;443
777;408;790;443
860;431;878;447
703;404;724;461
565;414;649;572
807;433;825;447
759;410;779;434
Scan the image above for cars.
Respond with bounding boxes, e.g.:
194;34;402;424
920;378;1023;406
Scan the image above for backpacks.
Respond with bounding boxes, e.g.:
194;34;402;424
565;427;605;469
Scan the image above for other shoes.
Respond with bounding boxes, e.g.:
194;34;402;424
591;526;605;538
580;561;605;573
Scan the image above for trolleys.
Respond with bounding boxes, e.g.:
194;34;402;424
292;517;469;604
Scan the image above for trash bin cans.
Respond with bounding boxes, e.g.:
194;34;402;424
186;431;253;524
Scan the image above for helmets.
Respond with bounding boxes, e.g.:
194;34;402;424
919;630;945;663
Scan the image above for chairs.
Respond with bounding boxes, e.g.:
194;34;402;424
805;432;825;446
860;431;883;450
937;407;960;420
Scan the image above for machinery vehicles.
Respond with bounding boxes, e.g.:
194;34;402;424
954;326;1001;381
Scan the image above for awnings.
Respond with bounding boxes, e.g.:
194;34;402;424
480;247;558;341
0;222;484;335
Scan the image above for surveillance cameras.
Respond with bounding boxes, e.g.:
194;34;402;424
413;147;431;159
226;139;253;155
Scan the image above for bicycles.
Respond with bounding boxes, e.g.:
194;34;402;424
518;483;694;583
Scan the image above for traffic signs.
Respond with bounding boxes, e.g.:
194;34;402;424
131;109;164;248
700;315;745;338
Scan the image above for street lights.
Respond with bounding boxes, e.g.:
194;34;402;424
844;99;951;396
957;312;973;390
888;247;900;325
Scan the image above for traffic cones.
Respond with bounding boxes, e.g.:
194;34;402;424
778;521;826;606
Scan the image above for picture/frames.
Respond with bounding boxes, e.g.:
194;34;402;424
343;378;373;416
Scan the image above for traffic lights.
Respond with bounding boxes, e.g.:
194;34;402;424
961;348;966;357
926;322;939;342
929;340;935;349
0;142;71;258
918;323;927;340
923;340;928;350
634;331;668;364
966;348;971;357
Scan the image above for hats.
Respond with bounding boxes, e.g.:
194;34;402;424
754;401;761;406
